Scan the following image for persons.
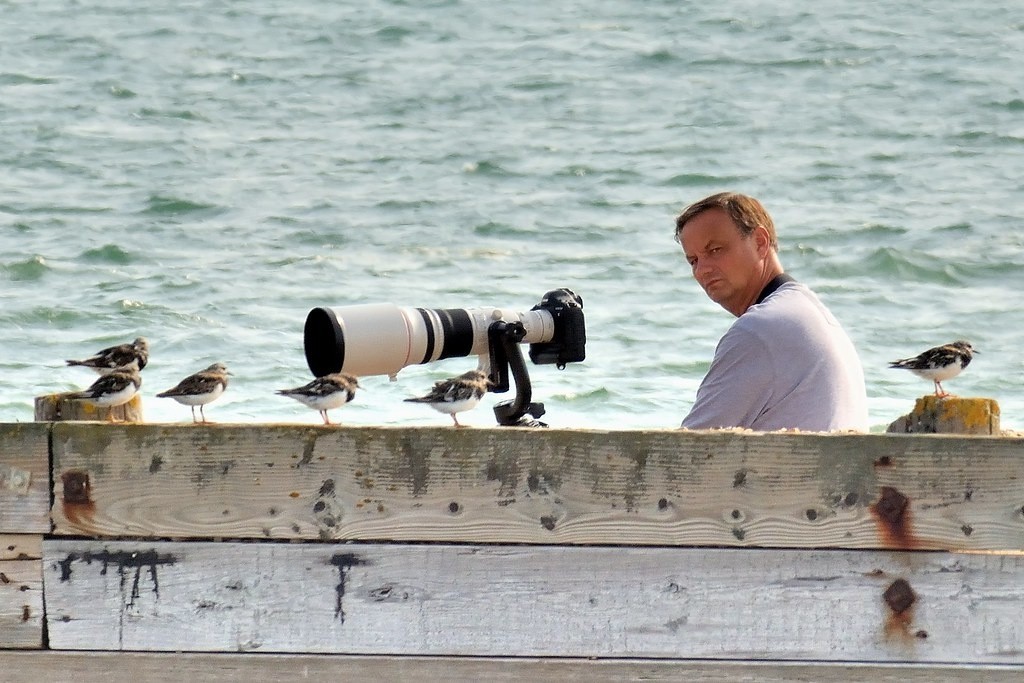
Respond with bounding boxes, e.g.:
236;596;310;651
674;192;868;431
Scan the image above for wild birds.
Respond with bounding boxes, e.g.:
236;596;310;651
402;369;498;430
886;340;982;400
64;337;151;426
274;373;367;426
155;363;235;425
